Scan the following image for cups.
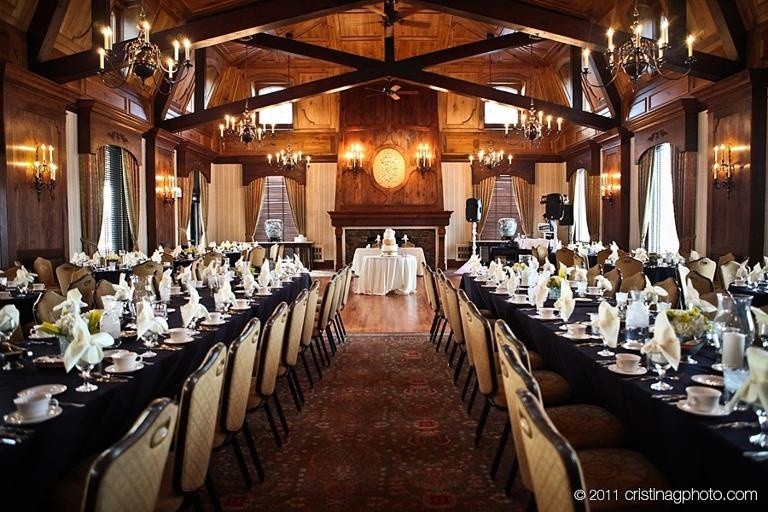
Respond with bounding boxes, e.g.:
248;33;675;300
206;312;224;322
282;276;292;280
168;328;191;342
587;287;598;293
538;308;553;317
495;287;507;291
722;332;744;369
685;386;722;412
12;393;59;418
0;291;12;296
615;353;641;372
232;299;250;308
33;284;45;289
196;281;203;286
569;281;576;285
735;280;744;285
29;324;53;336
163;260;172;266
110;352;143;370
567;323;587;336
270;279;282;287
258;287;268;293
512;294;526;301
171;286;181;293
485;280;496;285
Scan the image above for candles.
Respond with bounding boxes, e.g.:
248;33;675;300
377;234;380;240
404;234;407;239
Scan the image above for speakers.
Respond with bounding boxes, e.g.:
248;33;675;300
544;192;563;222
465;198;484;224
559;204;573;225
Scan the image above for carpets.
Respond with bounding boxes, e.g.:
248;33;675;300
182;331;538;512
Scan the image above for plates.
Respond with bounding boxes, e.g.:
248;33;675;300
268;285;284;288
731;284;748;287
229;306;251;310
254;293;272;296
32;289;47;290
279;281;294;282
563;333;591;340
104;363;144;373
608;363;647;375
569;286;578;287
508;300;529;304
170;292;184;295
3;406;63;425
493;291;509;294
200;320;226;325
16;383;67;397
28;334;55;339
691;374;724;386
534;315;556;319
480;284;498;287
103;349;129;357
584;293;601;295
676;399;731;417
0;296;13;300
163;337;194;344
195;286;206;287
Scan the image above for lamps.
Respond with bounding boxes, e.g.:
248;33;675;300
713;144;735;200
267;31;310;171
600;171;619;208
583;0;697;93
219;44;274;144
416;144;433;178
514;38;562;145
346;143;363;175
164;176;175;209
96;0;192;94
33;143;57;201
468;32;512;174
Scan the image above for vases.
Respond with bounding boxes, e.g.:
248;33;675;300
264;219;283;242
496;218;517;240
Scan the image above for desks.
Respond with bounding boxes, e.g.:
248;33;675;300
515;238;558;254
351;248;426;276
353;255;417;296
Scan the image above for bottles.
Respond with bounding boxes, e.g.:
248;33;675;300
713;292;755;354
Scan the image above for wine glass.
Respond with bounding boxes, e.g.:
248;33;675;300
559;311;573;330
597;329;614;357
222;303;232;318
618;302;627;318
141;329;157;358
650;352;675;391
191;317;201;335
75;358;98;393
249;288;255;302
0;328;24;371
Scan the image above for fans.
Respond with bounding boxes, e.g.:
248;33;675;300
364;78;417;100
351;0;432;28
